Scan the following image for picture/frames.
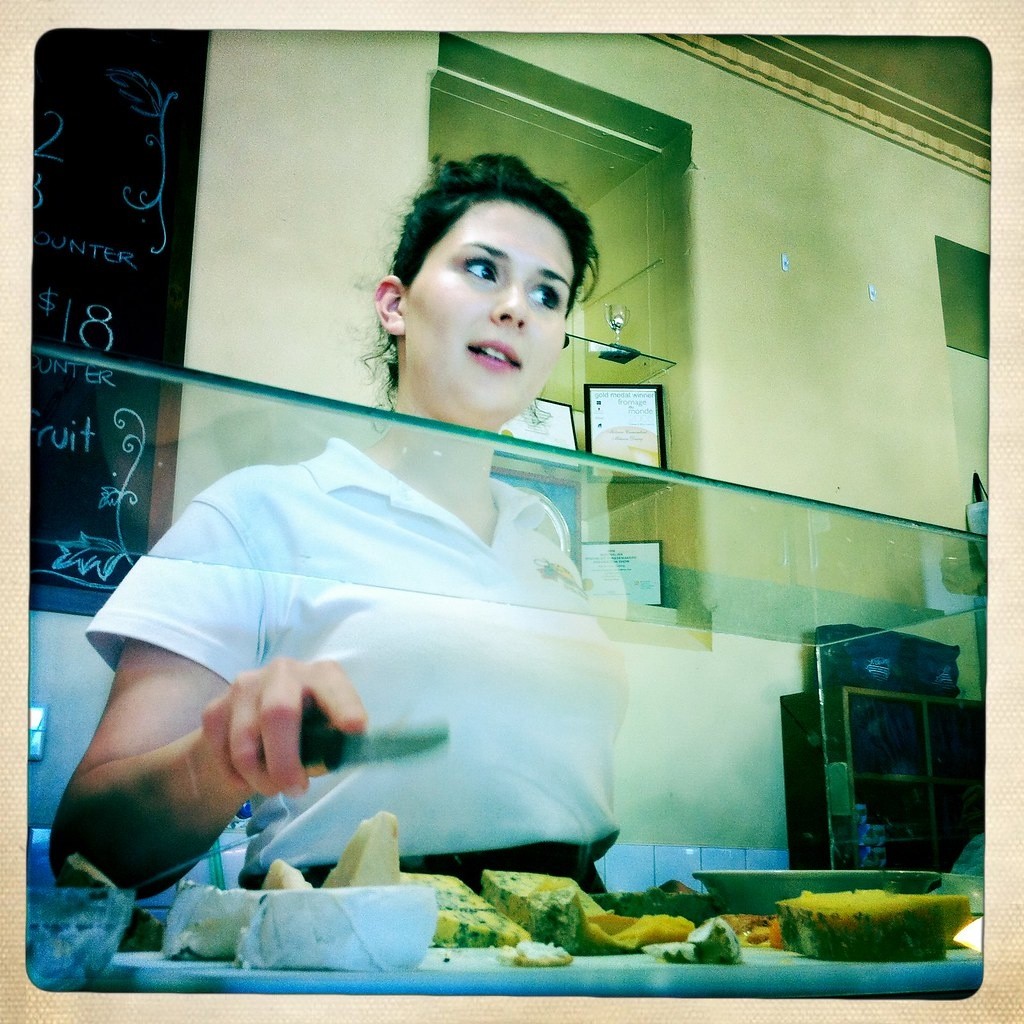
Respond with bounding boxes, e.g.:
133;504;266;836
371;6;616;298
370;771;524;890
583;383;668;485
493;397;581;473
490;465;582;575
580;540;664;608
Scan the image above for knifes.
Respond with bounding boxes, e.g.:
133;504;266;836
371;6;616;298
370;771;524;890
260;724;448;776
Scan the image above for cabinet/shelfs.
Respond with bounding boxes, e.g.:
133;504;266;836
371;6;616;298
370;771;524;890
781;685;985;872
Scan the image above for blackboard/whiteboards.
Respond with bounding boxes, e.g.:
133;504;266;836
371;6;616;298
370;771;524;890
29;29;213;618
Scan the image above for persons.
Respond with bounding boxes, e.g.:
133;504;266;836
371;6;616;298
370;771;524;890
50;155;621;914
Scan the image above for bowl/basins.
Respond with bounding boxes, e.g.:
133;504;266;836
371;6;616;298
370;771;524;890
25;885;135;992
691;870;944;914
